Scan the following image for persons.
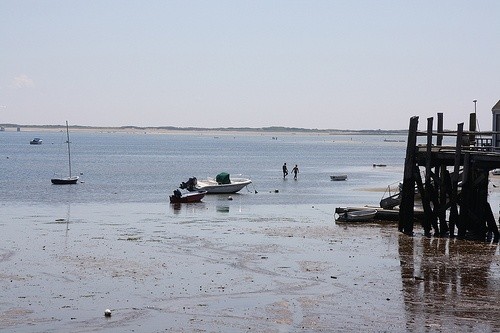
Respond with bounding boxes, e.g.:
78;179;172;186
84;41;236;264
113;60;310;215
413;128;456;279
291;164;299;180
282;162;288;178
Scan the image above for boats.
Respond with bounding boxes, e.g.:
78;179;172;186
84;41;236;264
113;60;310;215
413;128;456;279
334;164;500;225
330;174;347;181
30;137;43;144
179;172;253;194
168;187;208;204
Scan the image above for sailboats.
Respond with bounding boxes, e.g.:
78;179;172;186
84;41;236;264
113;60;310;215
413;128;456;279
51;120;80;185
